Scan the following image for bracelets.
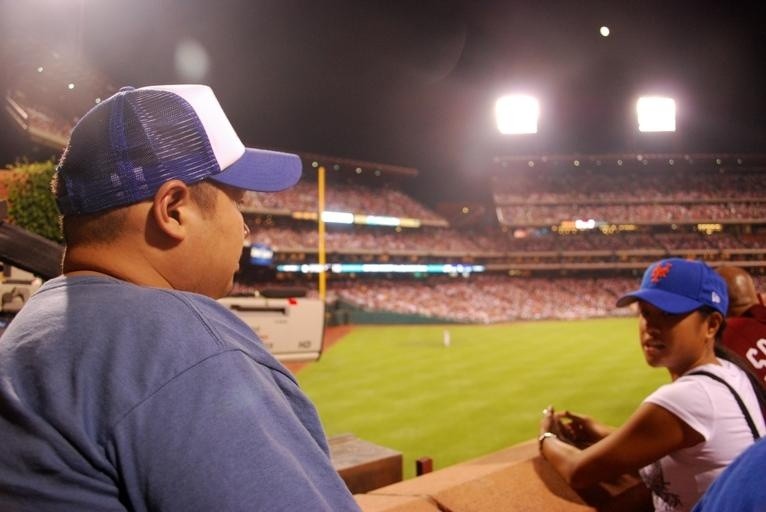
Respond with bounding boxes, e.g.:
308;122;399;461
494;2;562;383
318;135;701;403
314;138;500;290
538;432;557;459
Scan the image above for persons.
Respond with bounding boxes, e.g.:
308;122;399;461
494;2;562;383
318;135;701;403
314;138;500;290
226;169;766;324
536;258;766;512
0;82;366;512
714;266;766;388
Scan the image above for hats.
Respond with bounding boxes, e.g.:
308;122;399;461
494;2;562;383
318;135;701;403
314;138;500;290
614;258;731;316
50;82;305;219
